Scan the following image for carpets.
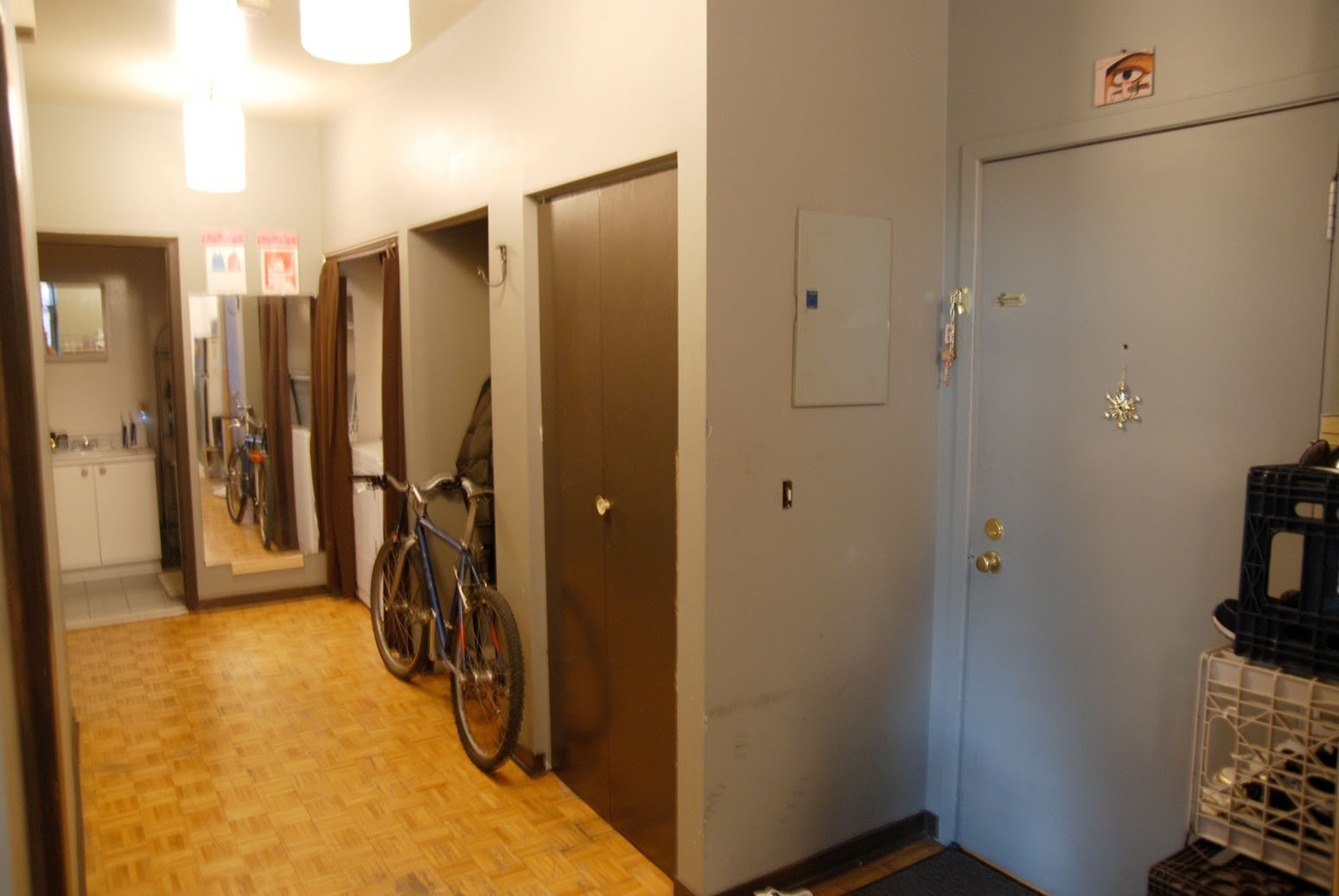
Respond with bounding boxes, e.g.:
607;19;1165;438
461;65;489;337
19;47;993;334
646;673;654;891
841;840;1049;896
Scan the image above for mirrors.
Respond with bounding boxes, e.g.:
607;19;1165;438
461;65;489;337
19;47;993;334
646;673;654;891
37;276;110;364
188;292;328;570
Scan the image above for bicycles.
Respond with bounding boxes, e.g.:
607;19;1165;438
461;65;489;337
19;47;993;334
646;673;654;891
225;403;275;550
348;474;525;773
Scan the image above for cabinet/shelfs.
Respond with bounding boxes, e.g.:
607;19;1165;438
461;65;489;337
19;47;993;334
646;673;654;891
52;461;163;585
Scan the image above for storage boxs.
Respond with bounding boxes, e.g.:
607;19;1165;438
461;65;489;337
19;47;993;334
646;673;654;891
1148;464;1339;896
